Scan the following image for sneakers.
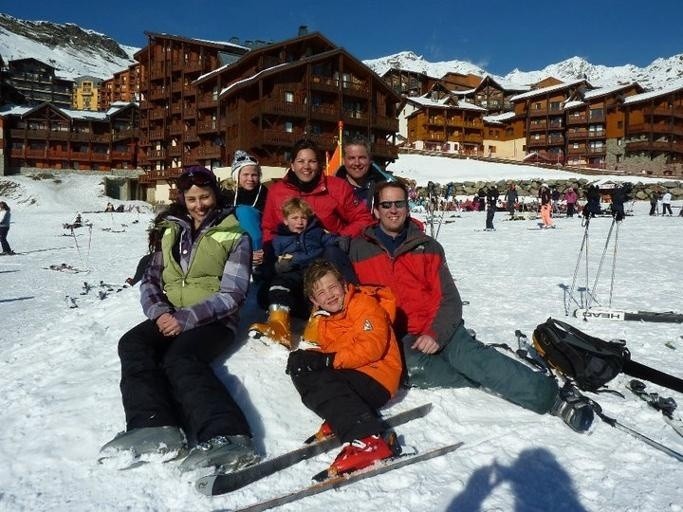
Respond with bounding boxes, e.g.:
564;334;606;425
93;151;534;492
305;421;335;445
548;386;594;434
311;428;403;483
98;425;189;472
178;433;260;478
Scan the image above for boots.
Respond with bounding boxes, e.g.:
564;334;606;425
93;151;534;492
302;310;330;349
247;303;294;351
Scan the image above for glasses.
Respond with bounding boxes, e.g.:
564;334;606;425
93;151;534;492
175;171;215;191
377;200;406;209
236;156;258;163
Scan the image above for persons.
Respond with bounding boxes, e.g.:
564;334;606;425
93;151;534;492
0;201;15;255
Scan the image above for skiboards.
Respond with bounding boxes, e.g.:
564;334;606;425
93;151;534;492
572;308;682;324
193;404;464;512
419;181;682;243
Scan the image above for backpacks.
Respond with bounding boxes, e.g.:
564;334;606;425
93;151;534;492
532;316;633;399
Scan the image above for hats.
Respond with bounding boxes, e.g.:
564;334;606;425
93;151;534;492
230;150;263;187
175;163;222;206
